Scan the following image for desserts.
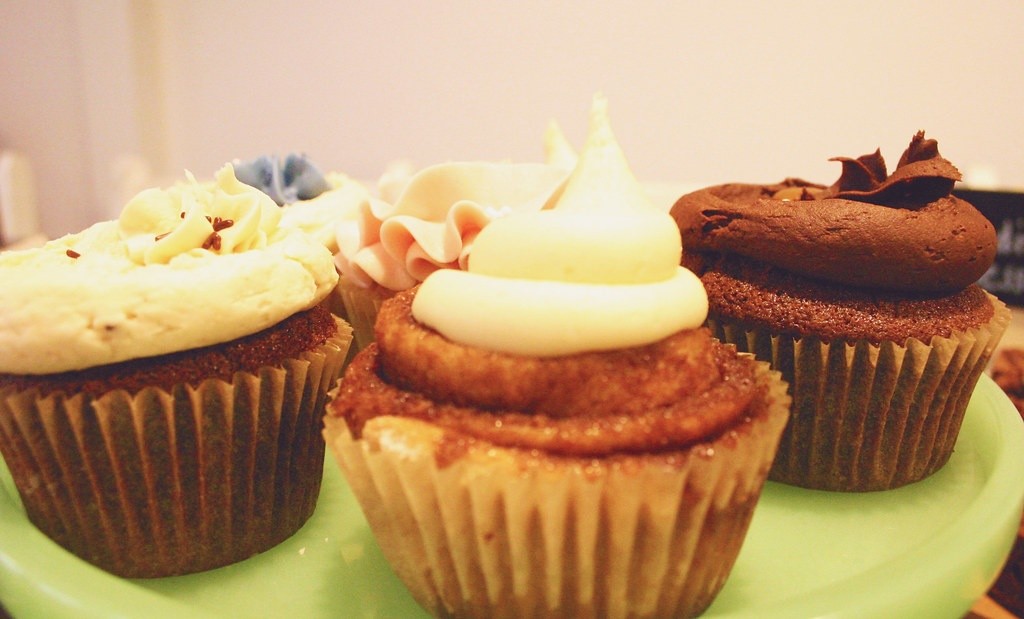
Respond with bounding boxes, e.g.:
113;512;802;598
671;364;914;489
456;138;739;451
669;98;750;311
0;93;1010;619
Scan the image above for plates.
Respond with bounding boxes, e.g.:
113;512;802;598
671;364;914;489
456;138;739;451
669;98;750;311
0;374;1024;619
